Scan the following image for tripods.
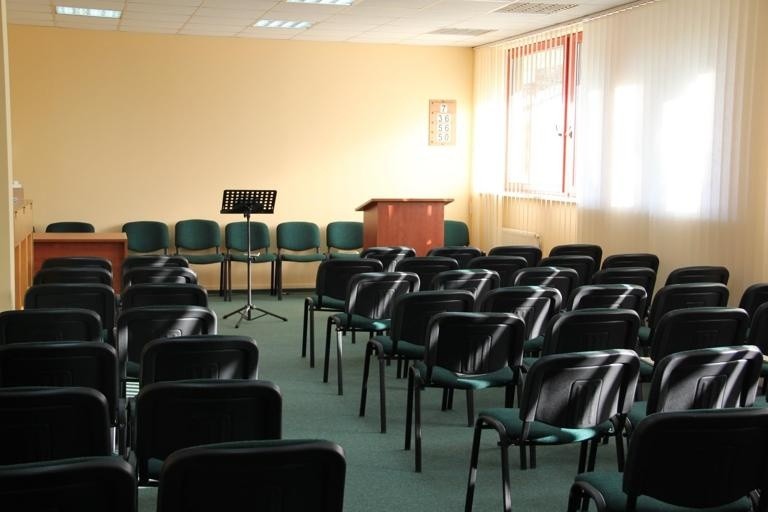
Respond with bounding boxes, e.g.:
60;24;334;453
222;213;288;330
